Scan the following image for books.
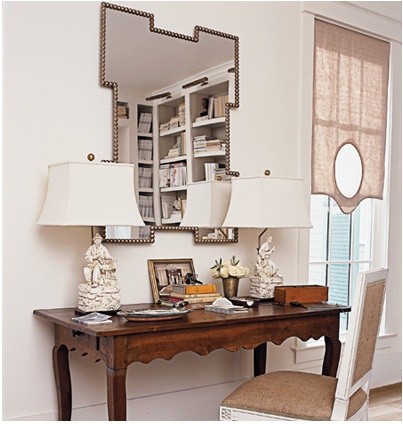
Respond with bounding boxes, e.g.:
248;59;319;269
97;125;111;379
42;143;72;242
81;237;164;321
139;140;153;162
301;302;333;309
222;227;233;239
161;283;221;310
193;93;229;123
159;163;186;188
138;195;153;219
70;311;112;325
139;113;153;134
161;195;187;224
193;134;225;154
204;163;225;181
203;295;248;314
159;102;185;134
161;132;186;160
139;225;150;239
137;167;153;190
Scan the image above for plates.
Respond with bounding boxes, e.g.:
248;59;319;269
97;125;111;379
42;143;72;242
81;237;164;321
116;307;192;322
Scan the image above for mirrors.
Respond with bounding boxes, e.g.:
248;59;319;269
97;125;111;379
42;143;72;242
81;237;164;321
99;2;238;245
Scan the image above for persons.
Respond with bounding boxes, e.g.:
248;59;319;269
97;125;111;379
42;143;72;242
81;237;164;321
82;232;111;288
258;234;283;277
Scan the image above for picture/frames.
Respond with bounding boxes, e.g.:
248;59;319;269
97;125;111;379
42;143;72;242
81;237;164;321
147;257;196;304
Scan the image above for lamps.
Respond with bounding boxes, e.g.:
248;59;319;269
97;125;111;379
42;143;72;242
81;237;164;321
33;153;146;317
179;180;233;239
220;169;314;298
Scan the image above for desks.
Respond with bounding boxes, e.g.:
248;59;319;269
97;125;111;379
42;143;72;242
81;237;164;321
33;301;351;421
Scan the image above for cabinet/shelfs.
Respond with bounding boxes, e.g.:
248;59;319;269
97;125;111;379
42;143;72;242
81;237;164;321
136;103;155;224
152;70;231;225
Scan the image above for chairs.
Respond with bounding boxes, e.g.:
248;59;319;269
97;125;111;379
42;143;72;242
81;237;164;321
219;268;392;421
